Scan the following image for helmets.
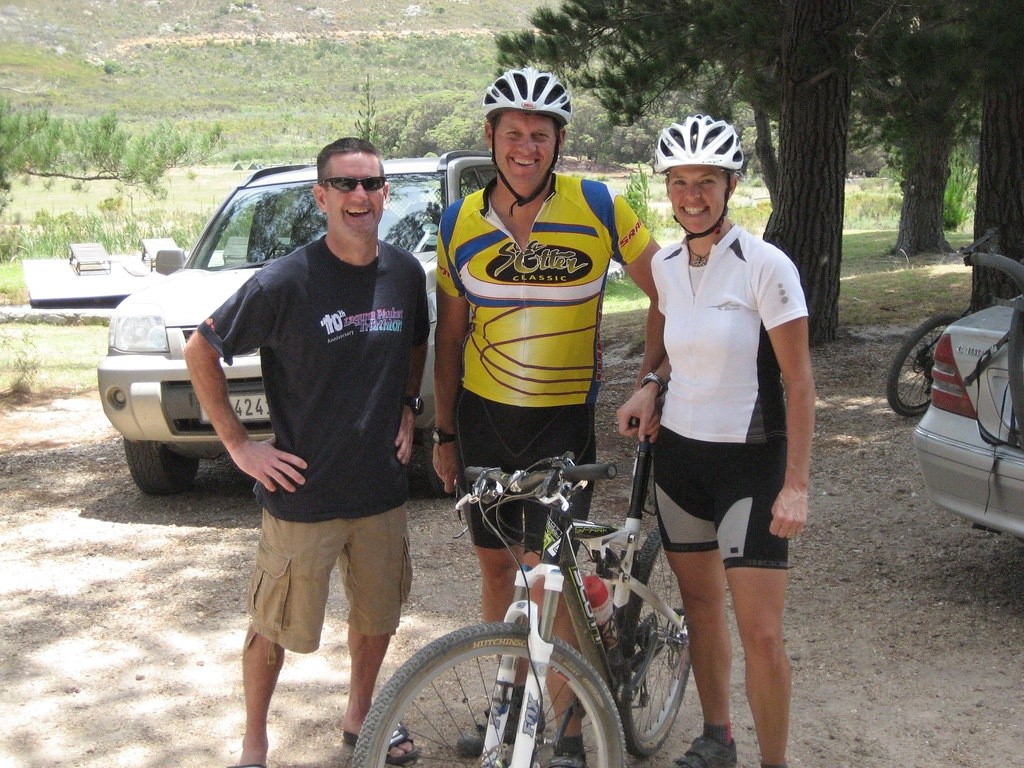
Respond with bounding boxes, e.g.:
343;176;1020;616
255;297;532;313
654;114;744;172
483;67;573;128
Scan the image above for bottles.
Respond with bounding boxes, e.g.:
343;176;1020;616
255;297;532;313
582;576;619;651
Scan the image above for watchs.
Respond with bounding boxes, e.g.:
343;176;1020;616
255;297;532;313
404;393;424;416
431;427;456;446
641;372;668;397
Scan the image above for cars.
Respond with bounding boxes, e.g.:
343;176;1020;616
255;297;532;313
912;262;1024;546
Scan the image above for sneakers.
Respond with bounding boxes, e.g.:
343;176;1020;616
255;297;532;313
673;735;737;768
457;707;546;754
546;750;586;768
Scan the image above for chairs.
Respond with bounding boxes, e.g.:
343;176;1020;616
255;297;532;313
68;243;113;275
275;237;291;256
223;237;249;265
141;238;181;272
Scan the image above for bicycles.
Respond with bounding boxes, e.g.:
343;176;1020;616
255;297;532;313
887;299;979;417
347;391;699;763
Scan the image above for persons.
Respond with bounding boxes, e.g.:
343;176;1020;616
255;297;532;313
614;114;817;768
430;67;666;768
181;137;431;767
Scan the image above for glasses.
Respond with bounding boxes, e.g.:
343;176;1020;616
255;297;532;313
318;176;386;192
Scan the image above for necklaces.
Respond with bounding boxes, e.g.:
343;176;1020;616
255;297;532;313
688;223;736;268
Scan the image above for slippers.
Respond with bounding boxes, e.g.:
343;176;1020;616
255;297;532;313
343;721;418;764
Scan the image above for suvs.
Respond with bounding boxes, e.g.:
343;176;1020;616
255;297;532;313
96;149;507;497
